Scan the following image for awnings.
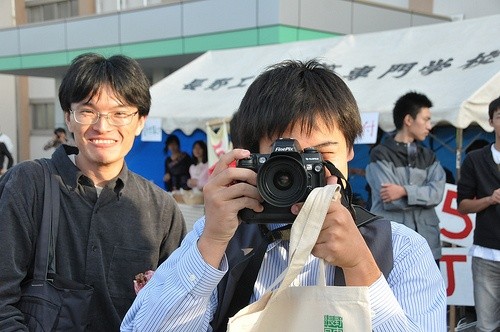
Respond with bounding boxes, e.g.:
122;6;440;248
141;14;500;143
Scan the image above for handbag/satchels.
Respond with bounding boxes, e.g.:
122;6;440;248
15;158;95;332
227;184;372;332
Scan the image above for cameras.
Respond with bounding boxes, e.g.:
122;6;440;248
237;137;327;224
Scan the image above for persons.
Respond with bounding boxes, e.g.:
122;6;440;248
186;140;210;191
0;52;188;332
120;59;447;332
44;128;73;151
163;133;194;192
0;142;14;176
457;96;500;332
365;92;446;270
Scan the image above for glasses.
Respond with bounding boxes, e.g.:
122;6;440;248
68;108;138;125
